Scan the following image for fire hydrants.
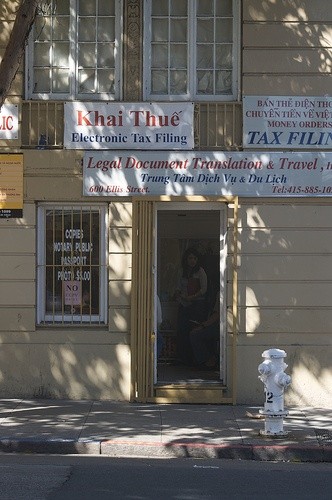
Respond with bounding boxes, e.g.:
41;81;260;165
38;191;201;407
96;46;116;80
258;349;291;436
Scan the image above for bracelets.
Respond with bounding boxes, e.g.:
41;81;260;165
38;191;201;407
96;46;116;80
201;322;207;329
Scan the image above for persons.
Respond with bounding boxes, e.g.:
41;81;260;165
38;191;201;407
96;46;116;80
71;284;100;316
189;289;220;371
167;246;208;365
155;295;165;360
